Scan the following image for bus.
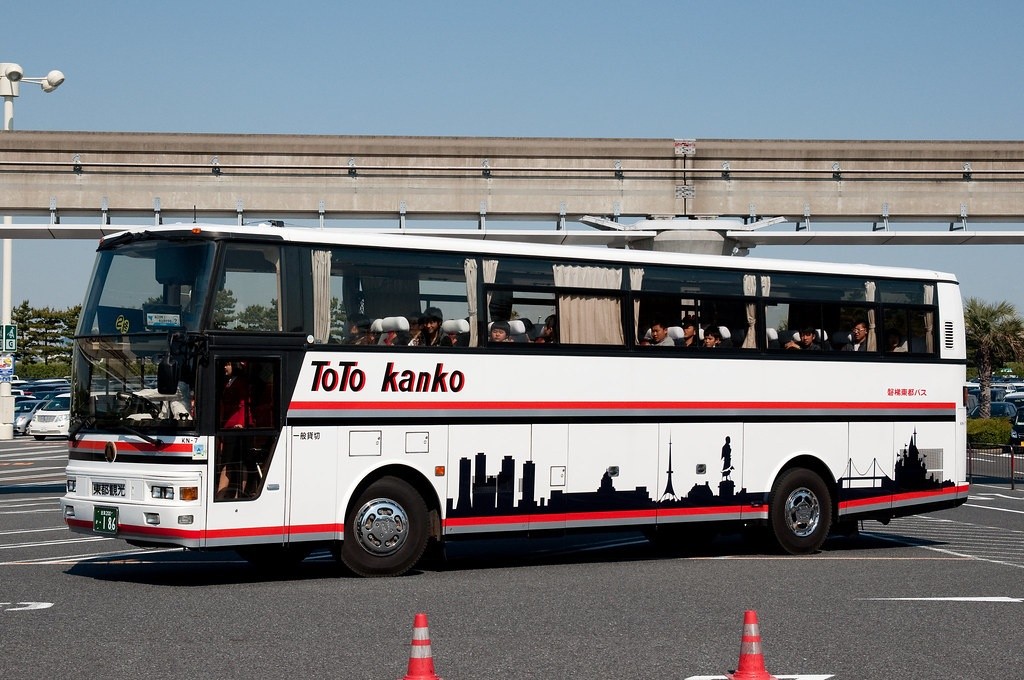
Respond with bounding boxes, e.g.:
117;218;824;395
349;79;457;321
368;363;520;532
57;219;970;578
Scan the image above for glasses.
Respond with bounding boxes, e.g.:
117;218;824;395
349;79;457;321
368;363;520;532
853;328;865;333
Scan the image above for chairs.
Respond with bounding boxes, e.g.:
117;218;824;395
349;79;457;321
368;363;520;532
371;317;908;353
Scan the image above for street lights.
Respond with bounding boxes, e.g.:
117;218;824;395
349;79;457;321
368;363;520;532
0;61;66;442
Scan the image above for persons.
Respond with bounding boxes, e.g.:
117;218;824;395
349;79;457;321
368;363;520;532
339;313;375;345
216;361;253;500
886;328;903;352
841;322;868;350
703;325;721;347
519;314;555;343
784;326;820;350
650;320;675;346
404;307;453;346
682;319;697;346
133;381;192;420
489;320;513;342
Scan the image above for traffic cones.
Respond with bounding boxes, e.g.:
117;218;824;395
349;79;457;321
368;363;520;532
402;614;444;680
724;610;779;680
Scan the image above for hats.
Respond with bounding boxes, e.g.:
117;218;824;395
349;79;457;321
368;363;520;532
681;318;698;329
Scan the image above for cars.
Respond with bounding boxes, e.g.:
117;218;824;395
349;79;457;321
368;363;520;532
10;375;194;442
17;349;72;364
967;374;1024;456
